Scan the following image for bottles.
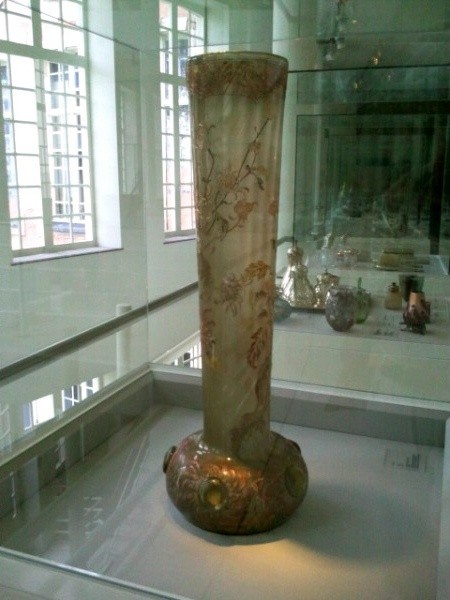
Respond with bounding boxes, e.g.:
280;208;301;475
384;282;402;312
